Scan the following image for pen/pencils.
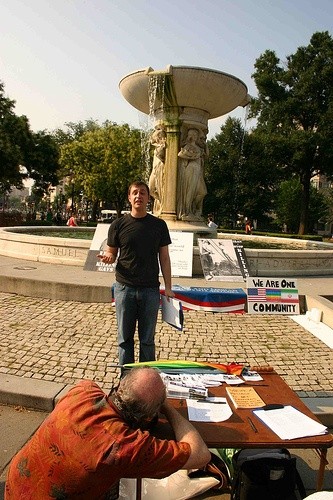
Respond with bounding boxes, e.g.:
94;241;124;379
167;376;223;399
246;416;257;433
197;401;226;404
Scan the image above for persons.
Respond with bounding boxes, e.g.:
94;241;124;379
147;129;167;215
178;129;207;221
5;368;211;500
67;213;78;227
208;217;218;229
96;181;176;379
245;221;253;235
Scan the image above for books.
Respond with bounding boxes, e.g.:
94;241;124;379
225;386;267;410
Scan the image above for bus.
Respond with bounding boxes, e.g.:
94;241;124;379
100;209;133;221
78;209;99;220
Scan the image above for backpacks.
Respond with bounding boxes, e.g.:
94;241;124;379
231;448;307;500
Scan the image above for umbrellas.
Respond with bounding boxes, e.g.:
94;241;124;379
120;359;274;376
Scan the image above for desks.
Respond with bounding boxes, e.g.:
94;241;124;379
132;367;333;500
